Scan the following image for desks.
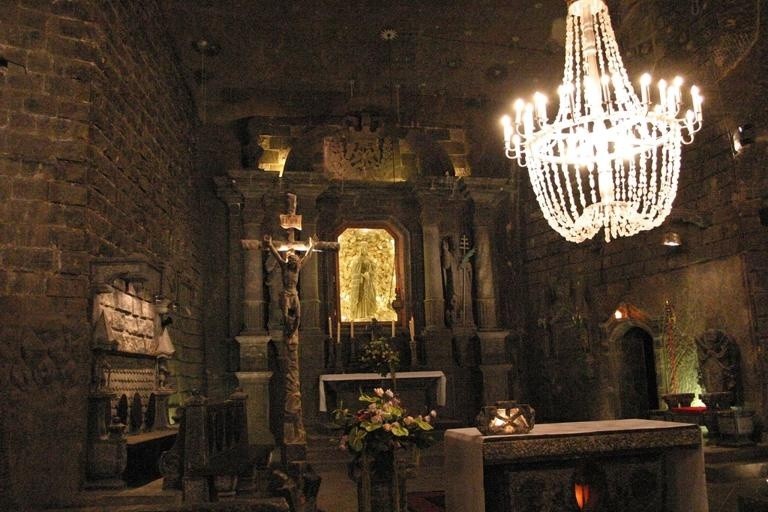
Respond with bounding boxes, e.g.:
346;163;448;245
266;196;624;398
319;371;446;412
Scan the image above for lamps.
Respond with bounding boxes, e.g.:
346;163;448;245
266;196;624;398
498;0;705;245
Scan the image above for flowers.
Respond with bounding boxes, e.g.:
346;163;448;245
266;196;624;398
330;386;437;477
362;341;401;379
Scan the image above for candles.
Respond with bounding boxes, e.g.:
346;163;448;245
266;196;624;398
392;320;395;337
329;317;332;338
337;319;340;343
409;320;414;341
351;320;354;338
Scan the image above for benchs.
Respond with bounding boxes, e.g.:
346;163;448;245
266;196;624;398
184;392;275;502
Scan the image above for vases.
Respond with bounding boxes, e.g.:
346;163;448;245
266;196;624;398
357;479;408;512
